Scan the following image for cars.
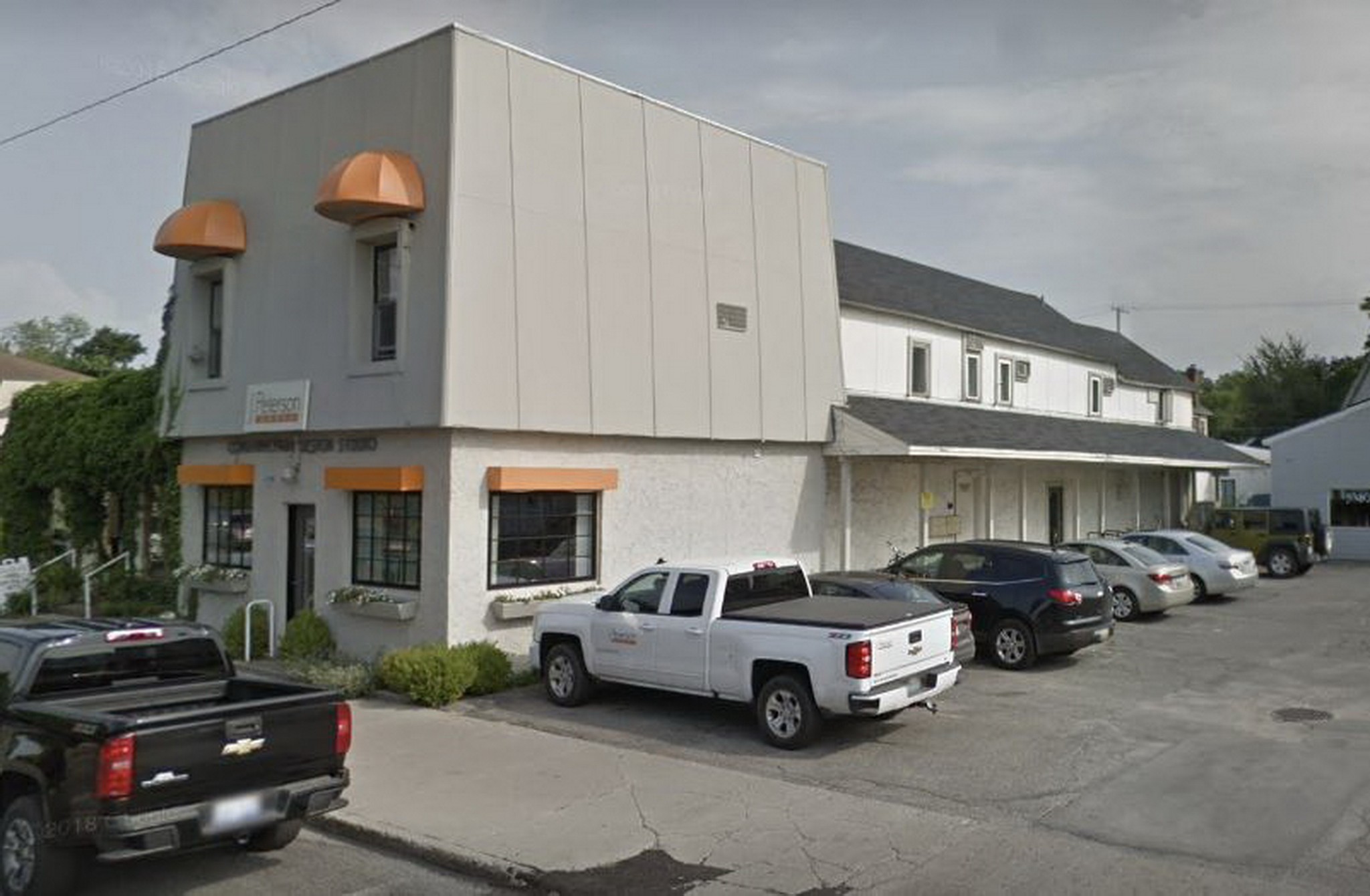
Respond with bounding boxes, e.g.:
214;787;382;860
808;563;979;667
1128;525;1260;596
868;539;1114;665
1057;537;1199;622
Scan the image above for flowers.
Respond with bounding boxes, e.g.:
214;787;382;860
494;587;603;605
323;585;404;606
168;564;248;584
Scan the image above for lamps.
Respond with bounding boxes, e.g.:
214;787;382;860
279;433;303;481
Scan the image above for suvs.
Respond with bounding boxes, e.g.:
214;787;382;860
1188;504;1331;576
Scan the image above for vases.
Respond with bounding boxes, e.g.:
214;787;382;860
184;580;249;593
330;602;414;620
493;592;605;619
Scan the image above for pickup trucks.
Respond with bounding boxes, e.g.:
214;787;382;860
3;617;350;894
534;558;960;750
